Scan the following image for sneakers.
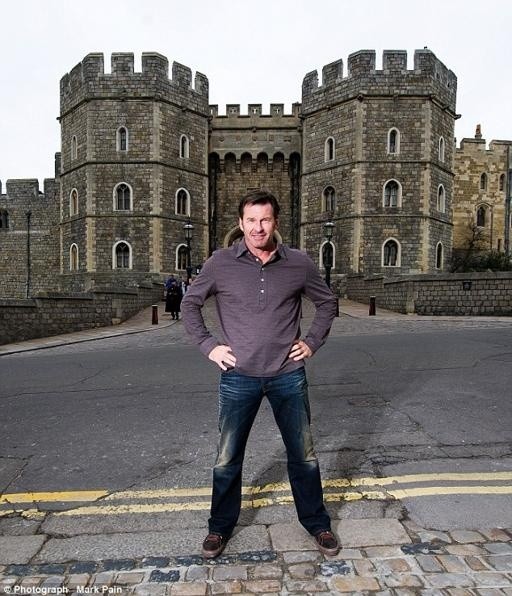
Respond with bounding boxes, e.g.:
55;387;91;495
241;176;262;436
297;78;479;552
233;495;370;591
202;534;225;558
313;530;339;556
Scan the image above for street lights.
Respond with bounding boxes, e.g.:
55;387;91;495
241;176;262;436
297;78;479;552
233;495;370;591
324;214;336;289
184;214;194;291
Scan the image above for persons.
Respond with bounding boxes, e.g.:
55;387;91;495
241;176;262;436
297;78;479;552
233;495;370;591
164;274;192;322
181;189;341;560
